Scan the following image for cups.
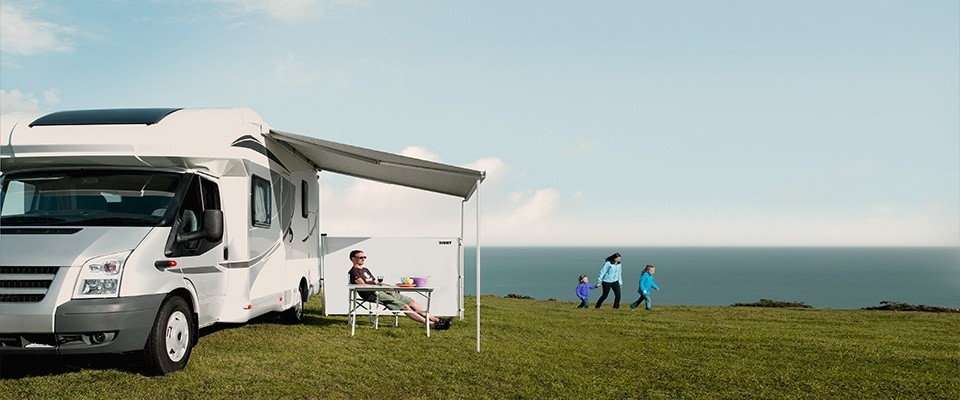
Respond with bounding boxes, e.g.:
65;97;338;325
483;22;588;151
406;280;413;285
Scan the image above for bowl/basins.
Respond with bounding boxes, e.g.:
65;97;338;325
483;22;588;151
401;277;409;283
411;277;427;287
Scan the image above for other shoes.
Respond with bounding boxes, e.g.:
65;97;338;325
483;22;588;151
434;317;454;331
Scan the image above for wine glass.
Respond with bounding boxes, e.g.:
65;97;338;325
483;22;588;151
377;276;384;286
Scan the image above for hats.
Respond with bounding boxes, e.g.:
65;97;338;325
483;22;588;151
578;274;589;281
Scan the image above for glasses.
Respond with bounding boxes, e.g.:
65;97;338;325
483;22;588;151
356;256;367;259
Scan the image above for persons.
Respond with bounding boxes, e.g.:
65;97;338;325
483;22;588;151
575;275;601;309
630;265;660;311
596;253;623;309
348;250;454;330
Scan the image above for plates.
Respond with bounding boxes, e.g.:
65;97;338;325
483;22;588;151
400;284;416;287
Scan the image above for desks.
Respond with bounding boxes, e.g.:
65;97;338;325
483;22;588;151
349;284;434;336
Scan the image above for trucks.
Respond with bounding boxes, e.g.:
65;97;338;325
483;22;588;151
0;105;324;376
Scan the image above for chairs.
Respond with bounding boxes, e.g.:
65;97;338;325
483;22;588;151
152;209;198;248
349;290;400;330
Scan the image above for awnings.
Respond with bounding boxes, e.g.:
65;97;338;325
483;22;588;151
269;129;486;353
